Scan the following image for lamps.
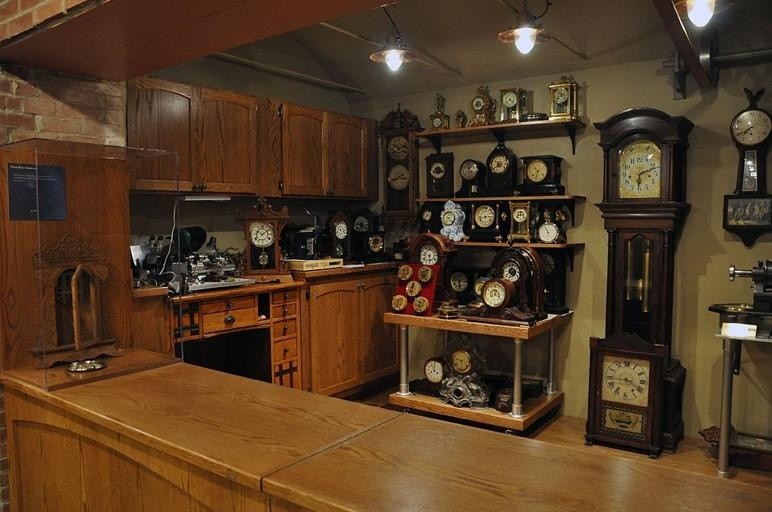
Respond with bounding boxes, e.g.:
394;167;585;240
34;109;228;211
369;7;419;72
497;0;554;55
674;0;735;28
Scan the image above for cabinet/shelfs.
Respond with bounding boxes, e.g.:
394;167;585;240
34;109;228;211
126;74;270;197
131;277;308;392
0;346;406;512
290;261;410;399
261;412;772;512
270;98;379;202
25;135;185;391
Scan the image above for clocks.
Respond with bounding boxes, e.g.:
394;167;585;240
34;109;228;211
722;87;772;249
458;278;537;327
235;197;295;283
584;336;688;460
428;92;451;132
424;151;455;199
486;140;518;197
505;200;540;244
466;200;511;243
498;80;530;124
538;208;569;245
347;207;377;265
592;105;695;340
536;247;575;315
548;75;582;121
424;333;491;410
518;154;566;197
446;266;474;305
455;158;486;198
489;241;548;323
325;209;353;265
464;83;498;129
380;102;427;232
440;199;466;242
366;232;385;259
418;204;436;235
410;233;461;314
471;278;487;306
